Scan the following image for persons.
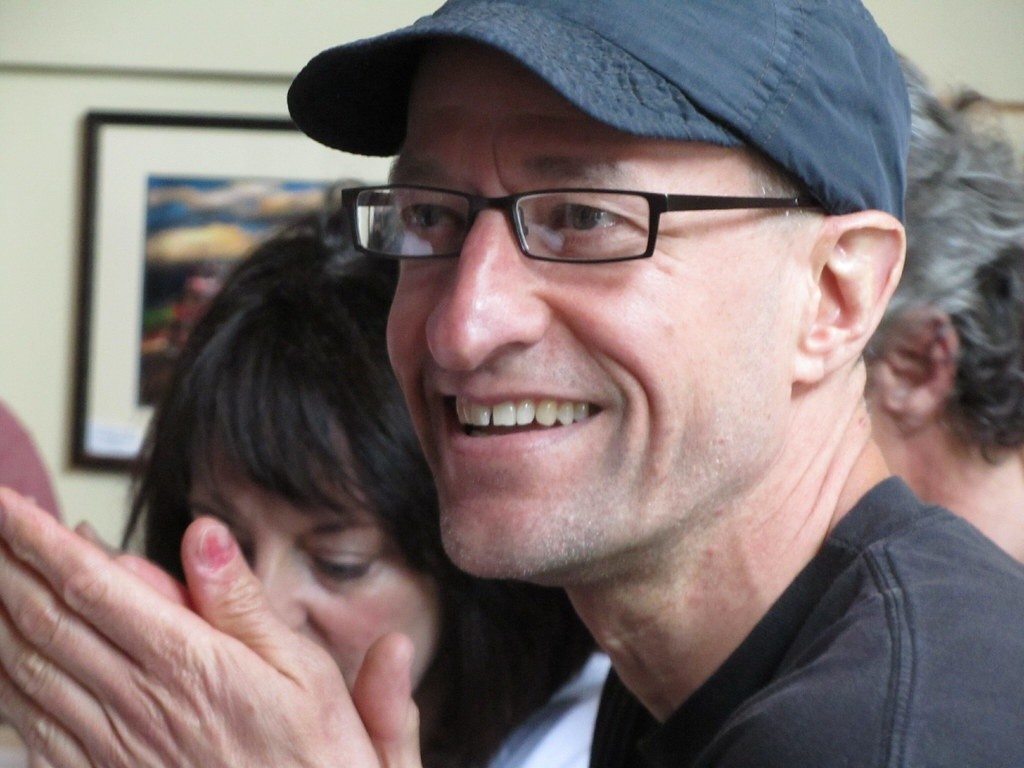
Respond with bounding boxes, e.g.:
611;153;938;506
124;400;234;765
119;206;611;768
862;52;1024;564
1;0;1024;766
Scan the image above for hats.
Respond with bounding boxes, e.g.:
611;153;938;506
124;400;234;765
288;0;912;224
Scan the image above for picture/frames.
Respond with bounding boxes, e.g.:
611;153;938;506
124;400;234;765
67;109;396;473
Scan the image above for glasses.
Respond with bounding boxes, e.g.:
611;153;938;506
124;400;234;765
339;176;817;263
